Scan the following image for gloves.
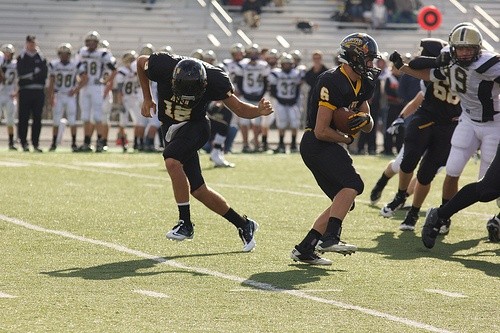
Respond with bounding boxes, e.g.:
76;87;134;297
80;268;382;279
345;131;361;145
390;51;404;71
386;116;405;135
347;111;370;130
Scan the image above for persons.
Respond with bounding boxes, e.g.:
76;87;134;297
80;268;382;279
138;51;274;252
216;0;283;27
291;33;382;265
327;0;423;30
371;21;500;250
0;30;422;155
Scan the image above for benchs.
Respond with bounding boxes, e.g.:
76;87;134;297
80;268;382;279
0;0;500;62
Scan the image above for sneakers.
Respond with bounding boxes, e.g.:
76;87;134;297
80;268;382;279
381;194;406;218
166;220;194;242
399;215;419;230
290;244;332;265
315;236;357;256
486;217;500;242
238;214;258;252
370;186;382;201
421;207;446;248
439;218;451;235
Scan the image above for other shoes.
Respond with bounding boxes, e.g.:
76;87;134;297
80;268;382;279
209;151;229;167
213;160;235;167
9;140;398;155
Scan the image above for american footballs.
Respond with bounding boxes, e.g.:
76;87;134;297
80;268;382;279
333;105;360;135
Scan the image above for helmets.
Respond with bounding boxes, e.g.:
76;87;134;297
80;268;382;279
2;44;15;56
337;32;382;86
449;23;473;44
171;59;207;109
57;43;72;55
85;30;414;74
449;26;482;68
